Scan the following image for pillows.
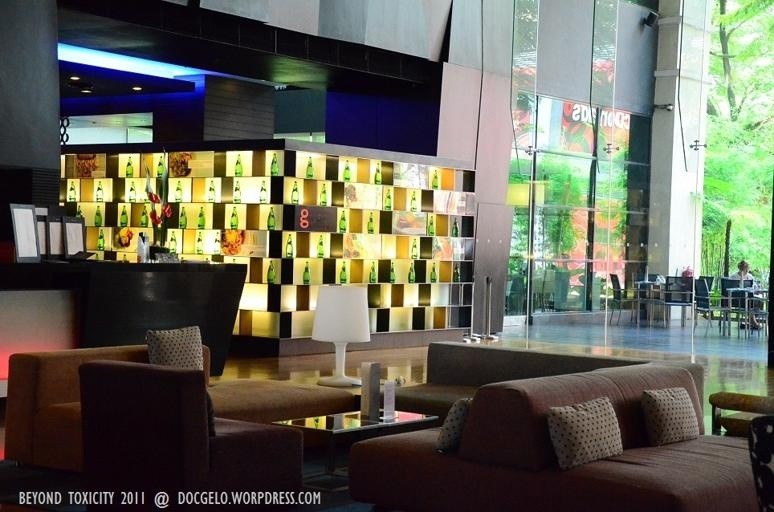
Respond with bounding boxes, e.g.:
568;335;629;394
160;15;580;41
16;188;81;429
547;394;624;471
147;325;203;370
435;398;475;452
642;386;701;447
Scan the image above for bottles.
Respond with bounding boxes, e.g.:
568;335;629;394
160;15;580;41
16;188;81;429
281;232;420;261
289;181;420;212
226;153;282;231
338;211;459;238
266;260;462;284
164;176;221;255
66;178;106;251
116;155;165;224
304;155;441;190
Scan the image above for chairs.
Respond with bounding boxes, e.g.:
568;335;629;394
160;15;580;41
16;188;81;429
506;266;768;340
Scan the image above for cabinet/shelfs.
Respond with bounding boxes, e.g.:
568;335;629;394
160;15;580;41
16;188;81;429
58;149;476;339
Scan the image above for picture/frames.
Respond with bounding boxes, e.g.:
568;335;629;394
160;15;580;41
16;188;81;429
10;204;59;265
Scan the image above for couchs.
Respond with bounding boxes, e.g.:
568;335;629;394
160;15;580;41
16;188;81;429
6;343;355;486
350;361;663;512
346;342;704;428
497;364;757;510
81;360;302;509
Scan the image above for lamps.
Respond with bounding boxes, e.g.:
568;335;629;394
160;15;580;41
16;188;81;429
641;11;659;27
312;285;371;388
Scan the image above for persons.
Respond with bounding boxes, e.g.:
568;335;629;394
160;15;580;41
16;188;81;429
729;260;762;329
